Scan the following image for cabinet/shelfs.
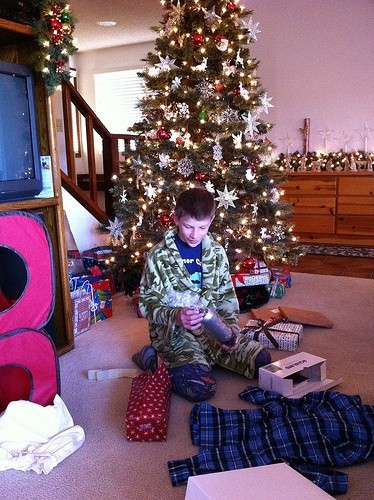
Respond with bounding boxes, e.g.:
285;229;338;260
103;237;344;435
274;170;374;258
0;18;79;359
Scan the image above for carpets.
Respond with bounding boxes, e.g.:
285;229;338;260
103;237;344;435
0;271;374;500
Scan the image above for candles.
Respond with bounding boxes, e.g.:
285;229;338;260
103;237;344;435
304;118;311;155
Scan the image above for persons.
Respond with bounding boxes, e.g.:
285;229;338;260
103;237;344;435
134;189;272;402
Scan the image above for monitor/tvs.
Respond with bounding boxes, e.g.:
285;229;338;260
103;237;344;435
0;61;43;204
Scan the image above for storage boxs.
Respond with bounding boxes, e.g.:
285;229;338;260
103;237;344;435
127;366;169;440
229;260;344;399
69;246;149;337
185;461;338;500
34;156;55;199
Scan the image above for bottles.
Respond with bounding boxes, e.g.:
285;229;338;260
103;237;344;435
198;307;237;347
